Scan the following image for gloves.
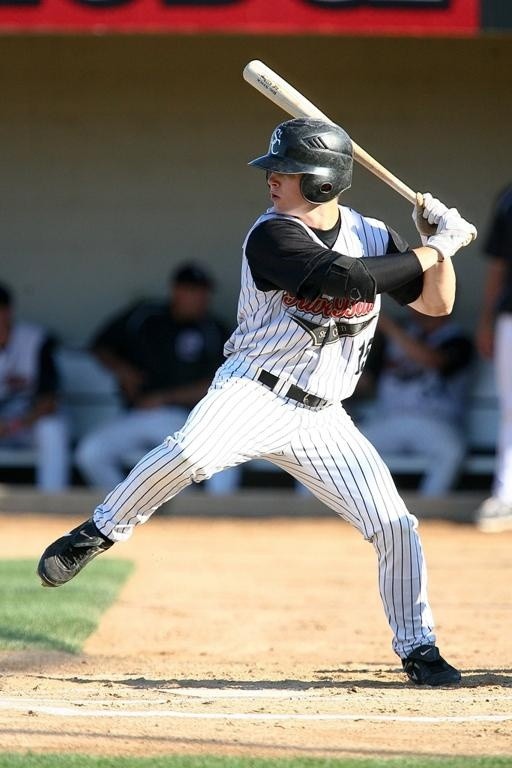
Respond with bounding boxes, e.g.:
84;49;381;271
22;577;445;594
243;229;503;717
426;207;478;263
411;192;449;246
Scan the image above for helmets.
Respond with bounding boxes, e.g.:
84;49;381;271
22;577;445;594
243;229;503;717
248;118;352;204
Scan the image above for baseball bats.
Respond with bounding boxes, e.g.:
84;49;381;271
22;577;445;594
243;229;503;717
242;60;477;247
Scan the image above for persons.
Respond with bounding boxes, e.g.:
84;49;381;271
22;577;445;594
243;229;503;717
75;260;240;493
357;305;477;494
473;185;512;533
38;118;478;684
0;281;74;492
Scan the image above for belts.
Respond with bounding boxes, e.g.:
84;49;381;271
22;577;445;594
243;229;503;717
258;370;326;407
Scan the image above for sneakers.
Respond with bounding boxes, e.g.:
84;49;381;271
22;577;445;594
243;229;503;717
38;518;114;586
402;644;460;685
477;499;510;519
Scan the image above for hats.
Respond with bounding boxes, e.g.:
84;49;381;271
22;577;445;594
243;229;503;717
179;266;214;290
